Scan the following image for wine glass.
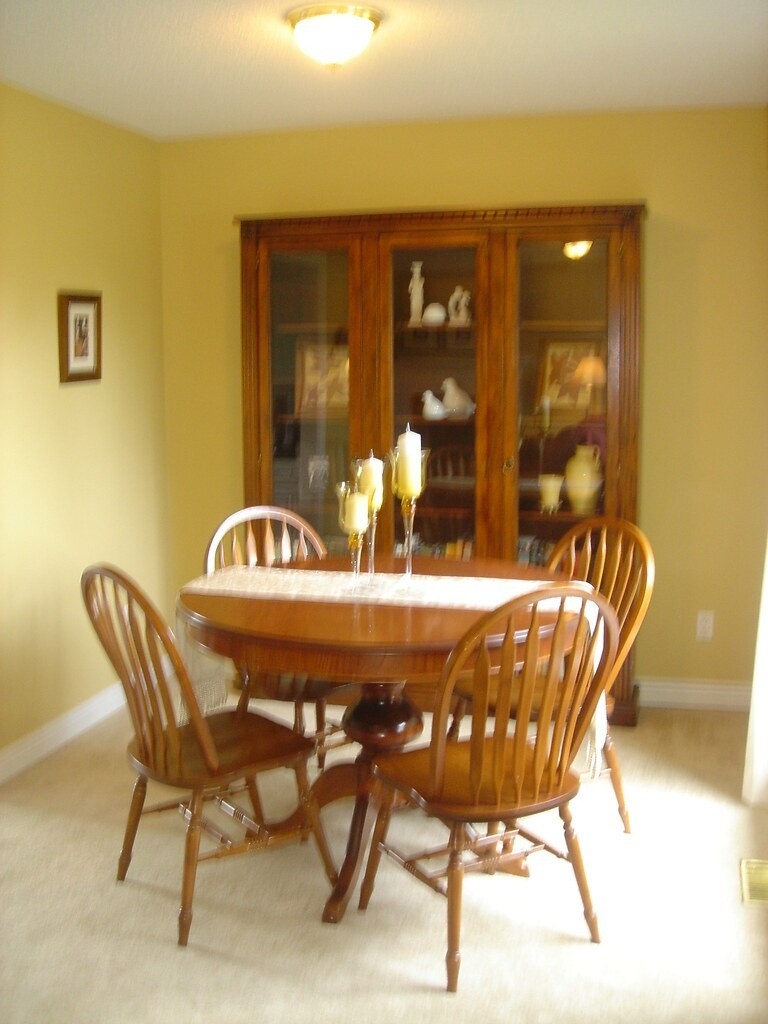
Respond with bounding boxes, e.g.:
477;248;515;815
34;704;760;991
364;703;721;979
388;446;430;584
334;480;376;595
348;458;391;589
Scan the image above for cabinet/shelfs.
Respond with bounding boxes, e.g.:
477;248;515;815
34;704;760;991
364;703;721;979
236;198;647;727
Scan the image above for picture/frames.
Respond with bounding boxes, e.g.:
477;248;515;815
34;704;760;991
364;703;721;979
532;338;605;419
292;336;350;420
56;292;102;381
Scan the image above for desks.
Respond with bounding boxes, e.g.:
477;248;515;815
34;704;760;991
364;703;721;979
181;556;584;873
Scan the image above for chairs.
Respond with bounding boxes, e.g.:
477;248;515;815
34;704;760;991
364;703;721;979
208;503;333;845
79;562;339;944
450;514;655;833
323;580;619;995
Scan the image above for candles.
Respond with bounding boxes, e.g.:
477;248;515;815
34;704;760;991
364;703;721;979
344;485;368;531
361;448;383;510
397;422;422;493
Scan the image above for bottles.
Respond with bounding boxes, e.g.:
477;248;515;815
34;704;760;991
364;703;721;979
565;444;603;516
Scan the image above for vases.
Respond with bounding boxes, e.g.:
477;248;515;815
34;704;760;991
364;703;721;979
566;443;602;517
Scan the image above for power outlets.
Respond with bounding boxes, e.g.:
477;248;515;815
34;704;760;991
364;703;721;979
697;610;713;638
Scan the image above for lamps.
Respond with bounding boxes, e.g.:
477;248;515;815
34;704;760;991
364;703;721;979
290;3;381;64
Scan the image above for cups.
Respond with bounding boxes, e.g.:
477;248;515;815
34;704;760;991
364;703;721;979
537;474;564;515
